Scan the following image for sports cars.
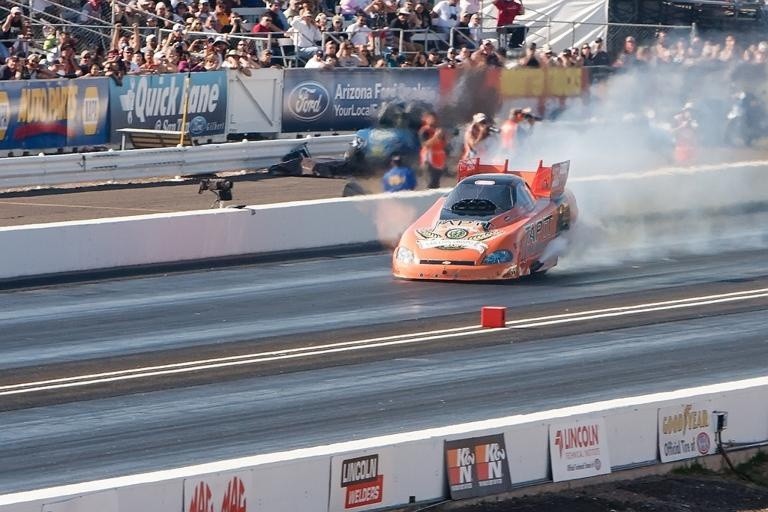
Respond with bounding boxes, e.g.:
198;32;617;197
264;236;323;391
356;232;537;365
391;157;579;281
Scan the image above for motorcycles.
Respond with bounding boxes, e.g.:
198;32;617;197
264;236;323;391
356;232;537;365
721;81;767;145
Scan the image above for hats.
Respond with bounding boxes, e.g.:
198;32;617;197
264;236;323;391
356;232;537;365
61;41;74;51
429;47;440;56
471;112;488;125
526;37;603;59
212;36;230;47
396;6;411;17
171;0;184;8
261;0;345;23
80;50;91;58
199;0;209;4
316;49;324;55
173;23;183;31
225;49;241;60
10;6;22;17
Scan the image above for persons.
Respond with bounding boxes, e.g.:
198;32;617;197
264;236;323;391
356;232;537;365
0;0;525;156
518;30;768;166
383;156;415;192
420;110;492;190
